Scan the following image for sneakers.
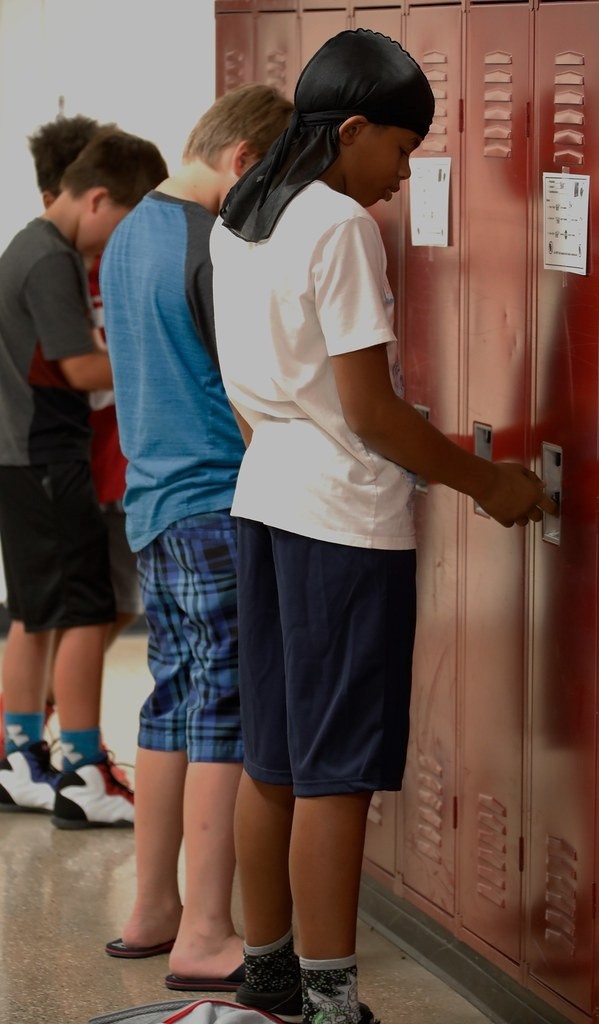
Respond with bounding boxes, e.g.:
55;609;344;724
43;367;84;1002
0;740;65;813
61;744;136;830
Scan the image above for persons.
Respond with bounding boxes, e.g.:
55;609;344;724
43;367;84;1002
0;133;169;830
207;29;560;1024
0;115;146;763
98;84;296;992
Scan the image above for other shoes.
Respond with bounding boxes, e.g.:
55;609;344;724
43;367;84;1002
235;977;376;1021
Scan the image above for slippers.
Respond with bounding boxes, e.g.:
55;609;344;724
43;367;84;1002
164;959;249;990
106;937;175;960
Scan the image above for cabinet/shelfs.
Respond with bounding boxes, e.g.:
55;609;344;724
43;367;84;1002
210;0;599;1022
